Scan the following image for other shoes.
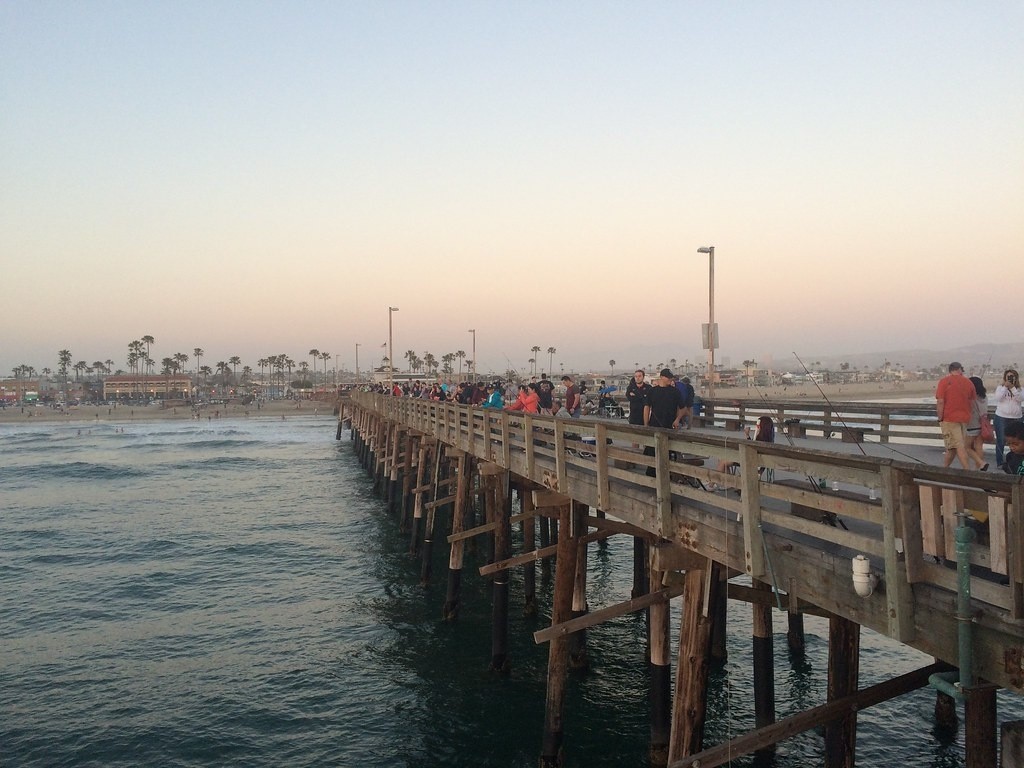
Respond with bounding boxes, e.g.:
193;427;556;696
980;464;989;471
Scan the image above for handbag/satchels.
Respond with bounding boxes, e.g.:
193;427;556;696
980;417;994;441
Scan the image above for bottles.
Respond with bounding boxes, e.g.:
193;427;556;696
819;478;826;488
868;488;876;499
831;480;840;491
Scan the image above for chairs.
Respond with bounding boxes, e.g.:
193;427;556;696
731;429;775;483
605;400;622;419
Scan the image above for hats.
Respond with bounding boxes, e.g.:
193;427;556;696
661;369;674;378
485;384;493;388
949;362;965;372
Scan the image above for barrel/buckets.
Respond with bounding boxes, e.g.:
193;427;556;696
582;436;596;458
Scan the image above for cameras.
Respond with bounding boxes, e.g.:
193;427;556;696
1008;375;1013;384
518;384;525;389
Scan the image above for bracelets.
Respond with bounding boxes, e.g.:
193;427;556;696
572;406;575;409
747;436;751;440
937;420;943;422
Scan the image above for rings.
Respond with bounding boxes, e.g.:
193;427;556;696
677;426;678;427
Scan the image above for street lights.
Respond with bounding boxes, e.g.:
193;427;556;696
468;329;475;383
697;247;714;427
389;307;399;396
356;343;361;384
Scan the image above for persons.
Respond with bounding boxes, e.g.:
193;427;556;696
336;384;351;391
504;373;556;452
709;416;774;488
552;376;597;439
355;378;503;445
626;368;695;478
598;380;626;419
935;362;1024;586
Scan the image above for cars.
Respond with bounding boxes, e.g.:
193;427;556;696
794;378;803;384
108;401;116;405
36;402;44;407
56;401;66;405
